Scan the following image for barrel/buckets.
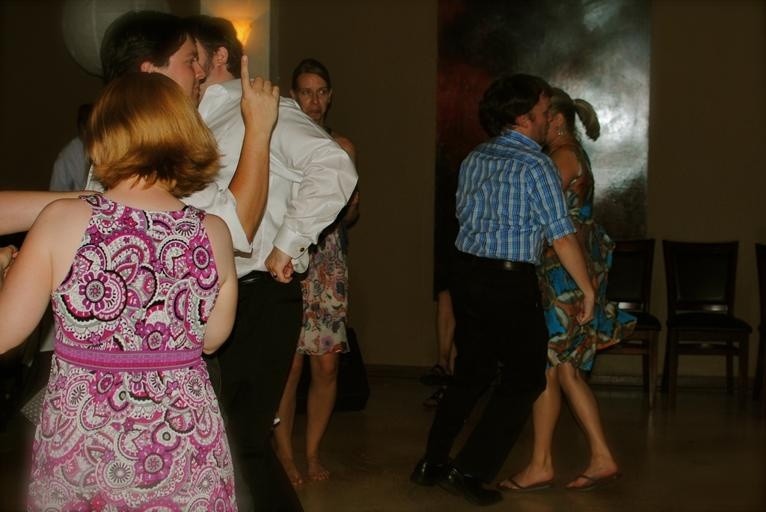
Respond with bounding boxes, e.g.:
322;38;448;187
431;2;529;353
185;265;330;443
497;478;552;492
421;366;455;406
566;473;622;491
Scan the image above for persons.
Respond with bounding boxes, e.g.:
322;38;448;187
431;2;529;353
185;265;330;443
421;146;463;407
184;15;358;511
274;61;359;486
0;71;239;512
87;13;281;254
410;74;594;502
1;138;100;235
497;87;618;493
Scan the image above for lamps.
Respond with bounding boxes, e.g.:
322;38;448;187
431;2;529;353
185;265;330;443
749;241;766;405
580;238;662;413
655;237;752;413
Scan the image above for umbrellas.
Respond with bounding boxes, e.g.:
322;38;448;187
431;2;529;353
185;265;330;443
410;460;501;505
